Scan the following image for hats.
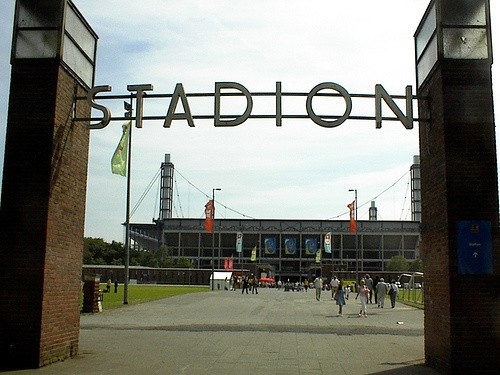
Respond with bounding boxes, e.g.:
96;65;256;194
380;278;384;281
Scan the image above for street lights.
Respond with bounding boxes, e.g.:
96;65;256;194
349;189;364;278
211;188;222;291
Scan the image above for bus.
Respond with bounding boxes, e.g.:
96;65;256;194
395;271;424;289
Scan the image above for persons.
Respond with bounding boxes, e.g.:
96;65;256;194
232;273;399;318
107;277;119;293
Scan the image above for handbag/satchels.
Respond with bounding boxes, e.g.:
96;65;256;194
388;287;394;296
